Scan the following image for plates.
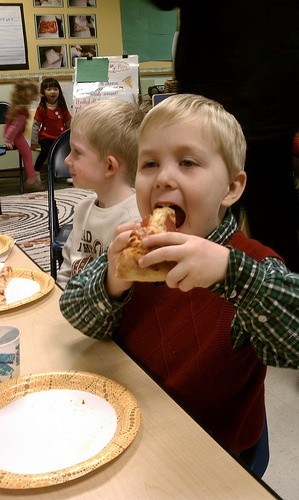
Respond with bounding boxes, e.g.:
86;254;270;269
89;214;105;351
0;267;59;312
0;370;142;489
0;234;16;254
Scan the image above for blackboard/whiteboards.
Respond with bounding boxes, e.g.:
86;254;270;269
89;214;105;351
73;55;142;116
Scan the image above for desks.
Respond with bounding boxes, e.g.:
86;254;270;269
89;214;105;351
0;242;284;500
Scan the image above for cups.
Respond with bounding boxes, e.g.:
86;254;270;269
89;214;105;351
0;325;21;382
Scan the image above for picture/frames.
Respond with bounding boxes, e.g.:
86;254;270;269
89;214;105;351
34;14;66;39
69;43;98;68
37;44;68;70
0;101;24;194
67;0;96;8
68;14;97;39
32;0;64;9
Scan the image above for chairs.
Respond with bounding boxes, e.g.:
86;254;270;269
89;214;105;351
48;129;73;279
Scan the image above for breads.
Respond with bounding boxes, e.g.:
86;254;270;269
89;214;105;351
0;234;14;254
0;265;13;296
116;206;177;281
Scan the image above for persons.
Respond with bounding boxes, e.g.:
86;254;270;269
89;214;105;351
56;98;143;289
31;78;73;173
59;93;299;481
146;0;299;272
3;80;45;191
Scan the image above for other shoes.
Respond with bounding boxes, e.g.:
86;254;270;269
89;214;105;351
23;179;45;192
